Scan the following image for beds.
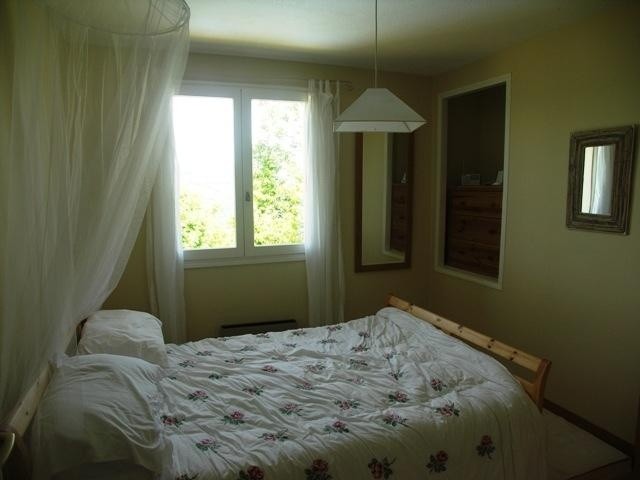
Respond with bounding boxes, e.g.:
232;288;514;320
31;296;549;479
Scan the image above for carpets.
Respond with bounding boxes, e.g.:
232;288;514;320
543;406;629;479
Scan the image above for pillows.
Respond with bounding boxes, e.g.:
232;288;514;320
30;353;165;480
75;309;169;369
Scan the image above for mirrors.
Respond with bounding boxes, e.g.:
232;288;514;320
434;73;511;291
565;123;638;236
355;132;414;273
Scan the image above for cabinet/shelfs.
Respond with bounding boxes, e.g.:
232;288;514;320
444;185;503;279
390;182;409;251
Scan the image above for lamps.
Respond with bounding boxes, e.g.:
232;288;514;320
332;0;428;134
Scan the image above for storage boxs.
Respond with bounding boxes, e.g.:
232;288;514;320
456;170;480;185
